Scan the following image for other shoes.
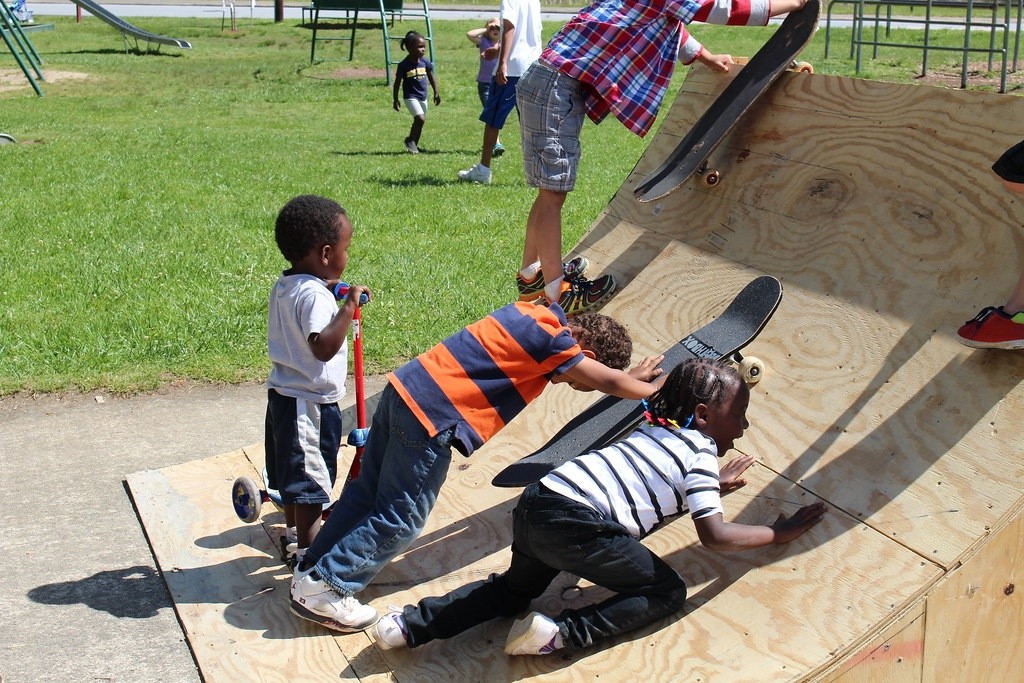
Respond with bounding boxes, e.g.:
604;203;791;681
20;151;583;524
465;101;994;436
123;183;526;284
281;536;303;573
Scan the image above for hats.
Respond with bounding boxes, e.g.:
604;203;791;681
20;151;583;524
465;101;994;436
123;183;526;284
485;18;500;31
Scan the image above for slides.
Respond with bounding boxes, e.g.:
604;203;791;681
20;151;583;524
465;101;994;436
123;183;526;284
73;0;193;49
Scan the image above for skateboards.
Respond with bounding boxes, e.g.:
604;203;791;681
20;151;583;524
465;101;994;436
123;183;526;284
629;0;824;202
491;276;785;488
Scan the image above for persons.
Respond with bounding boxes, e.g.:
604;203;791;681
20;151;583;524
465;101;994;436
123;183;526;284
289;302;668;633
264;194;371;565
466;19;505;157
370;356;828;654
457;0;543;185
955;140;1024;350
515;0;807;318
393;30;441;154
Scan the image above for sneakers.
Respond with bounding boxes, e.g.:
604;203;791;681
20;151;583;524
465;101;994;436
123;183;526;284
372;606;411;651
957;305;1024;350
290;571;377;634
505;612;560;657
458;165;493;186
543;273;616;320
405;137;419;154
518;255;589;303
289;559;321;602
493;145;505;155
